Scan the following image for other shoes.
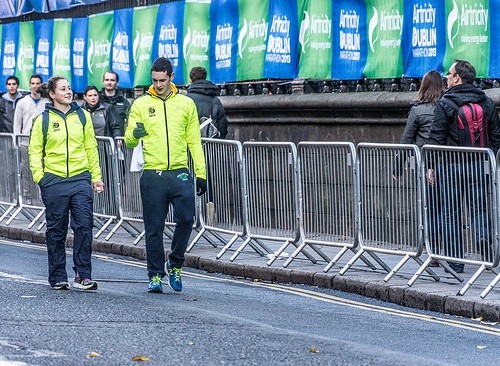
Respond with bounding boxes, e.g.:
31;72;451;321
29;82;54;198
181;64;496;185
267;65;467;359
478;237;494;270
444;262;465;273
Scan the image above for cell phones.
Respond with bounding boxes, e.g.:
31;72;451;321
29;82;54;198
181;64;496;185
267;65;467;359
136;122;145;130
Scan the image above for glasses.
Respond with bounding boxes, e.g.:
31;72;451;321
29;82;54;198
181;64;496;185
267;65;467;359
447;72;453;75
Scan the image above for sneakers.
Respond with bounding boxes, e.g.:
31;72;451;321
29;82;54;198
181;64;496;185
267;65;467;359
147;273;163;293
164;260;182;292
73;275;97;290
52;281;71;290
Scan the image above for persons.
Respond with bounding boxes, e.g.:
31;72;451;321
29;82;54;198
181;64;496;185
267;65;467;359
28;76;105;290
426;60;500;273
13;75;51;205
124;57;207;293
99;71;131;183
82;86;122;216
0;76;25;133
186;67;228;227
392;70;447;267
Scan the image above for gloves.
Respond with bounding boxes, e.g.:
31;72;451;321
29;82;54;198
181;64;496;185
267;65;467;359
133;124;148;139
196;177;207;196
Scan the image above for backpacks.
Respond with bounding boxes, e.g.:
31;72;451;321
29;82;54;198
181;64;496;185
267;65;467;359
199;116;222;162
440;94;491;147
